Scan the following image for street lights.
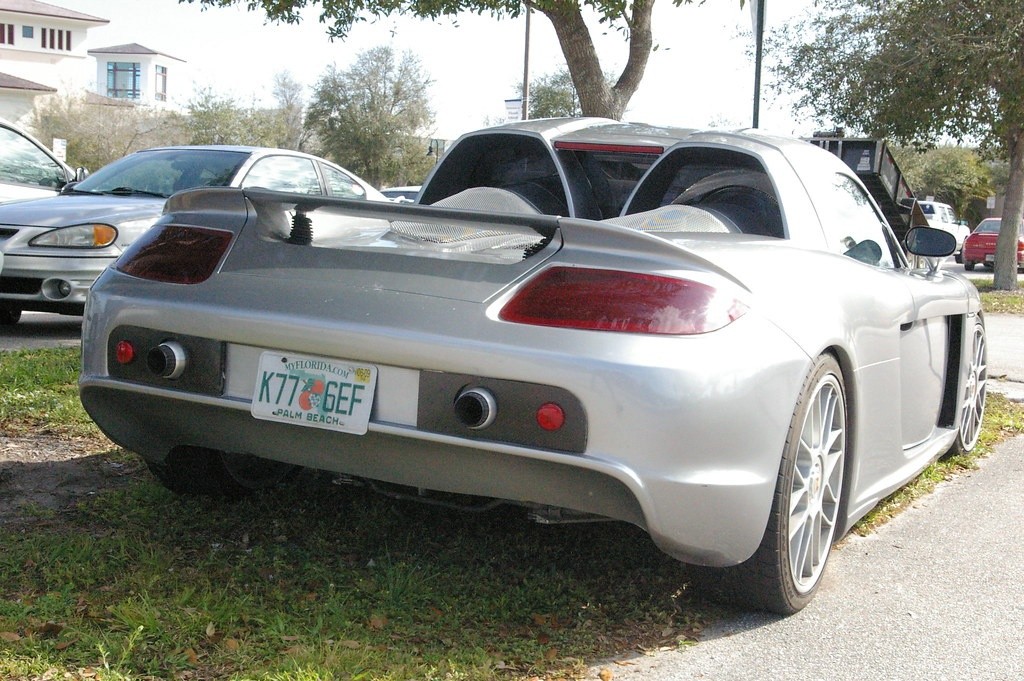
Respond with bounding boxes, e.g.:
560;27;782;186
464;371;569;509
426;138;439;163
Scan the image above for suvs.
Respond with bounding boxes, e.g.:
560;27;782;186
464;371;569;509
917;200;971;264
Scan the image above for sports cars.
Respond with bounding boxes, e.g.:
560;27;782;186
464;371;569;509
78;116;992;616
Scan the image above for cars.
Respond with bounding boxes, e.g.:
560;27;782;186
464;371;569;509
378;185;423;204
962;217;1024;273
0;144;405;324
0;116;90;204
